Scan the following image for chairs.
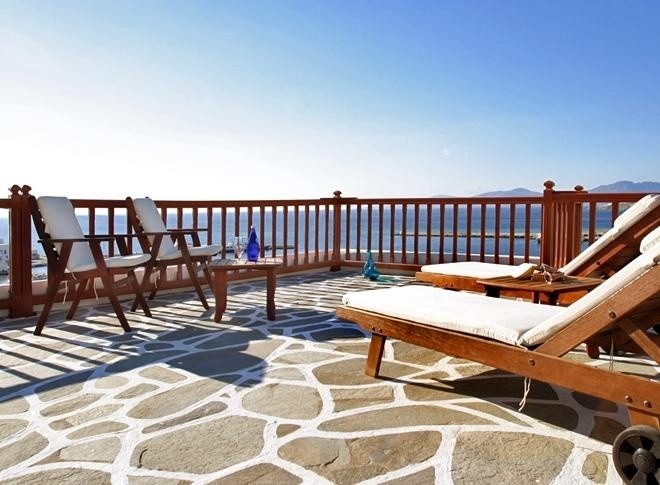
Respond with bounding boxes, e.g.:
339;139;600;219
333;228;660;483
413;194;660;366
25;195;226;335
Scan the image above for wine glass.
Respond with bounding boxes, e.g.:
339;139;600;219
232;237;245;265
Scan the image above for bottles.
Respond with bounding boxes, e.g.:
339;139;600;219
365;249;378;280
246;226;259;262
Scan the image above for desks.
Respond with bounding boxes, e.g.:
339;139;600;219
475;274;607;360
